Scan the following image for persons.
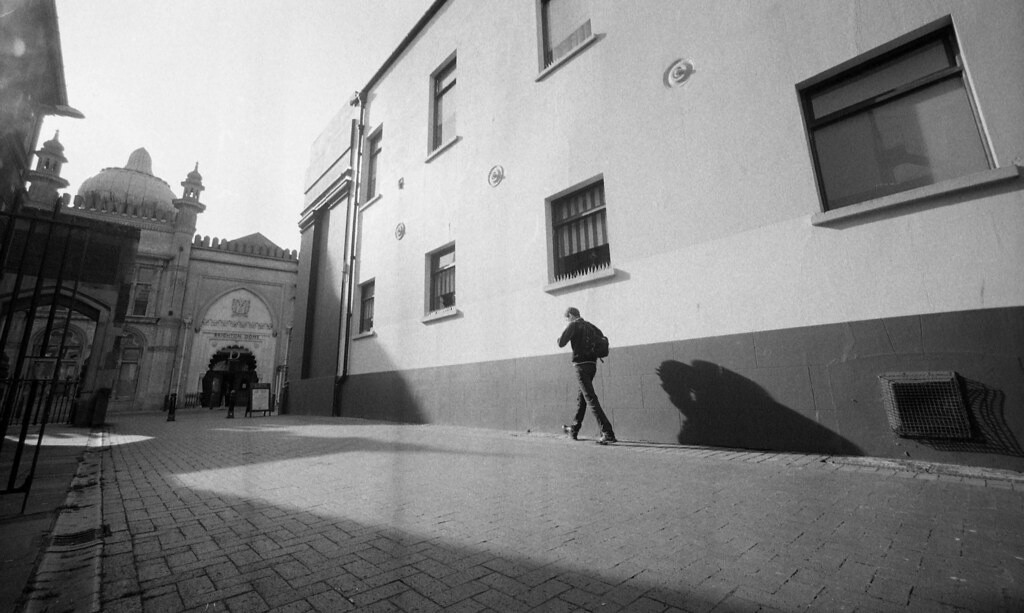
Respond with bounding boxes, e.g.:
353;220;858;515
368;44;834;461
557;307;617;445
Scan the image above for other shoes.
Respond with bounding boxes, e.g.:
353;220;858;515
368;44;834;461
595;434;618;445
562;424;578;439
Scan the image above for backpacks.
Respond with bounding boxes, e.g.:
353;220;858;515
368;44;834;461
574;319;609;363
